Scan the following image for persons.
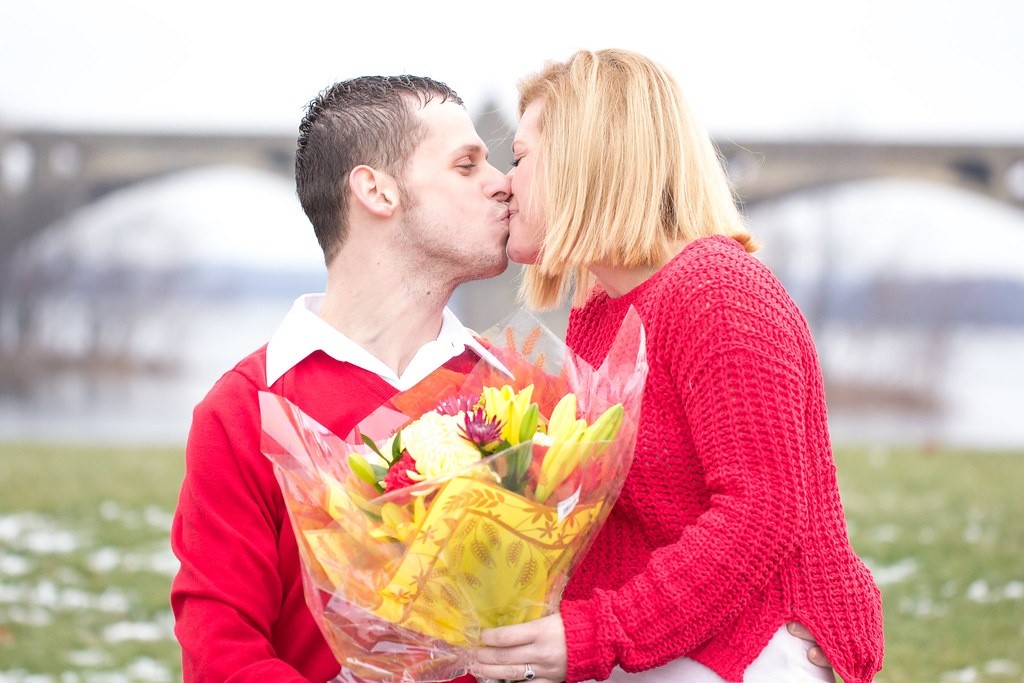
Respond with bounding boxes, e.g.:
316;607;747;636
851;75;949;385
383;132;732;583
169;75;833;683
471;49;884;683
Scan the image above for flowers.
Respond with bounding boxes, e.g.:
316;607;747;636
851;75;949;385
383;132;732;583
259;306;649;683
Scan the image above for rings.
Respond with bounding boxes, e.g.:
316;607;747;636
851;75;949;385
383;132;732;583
524;664;535;680
